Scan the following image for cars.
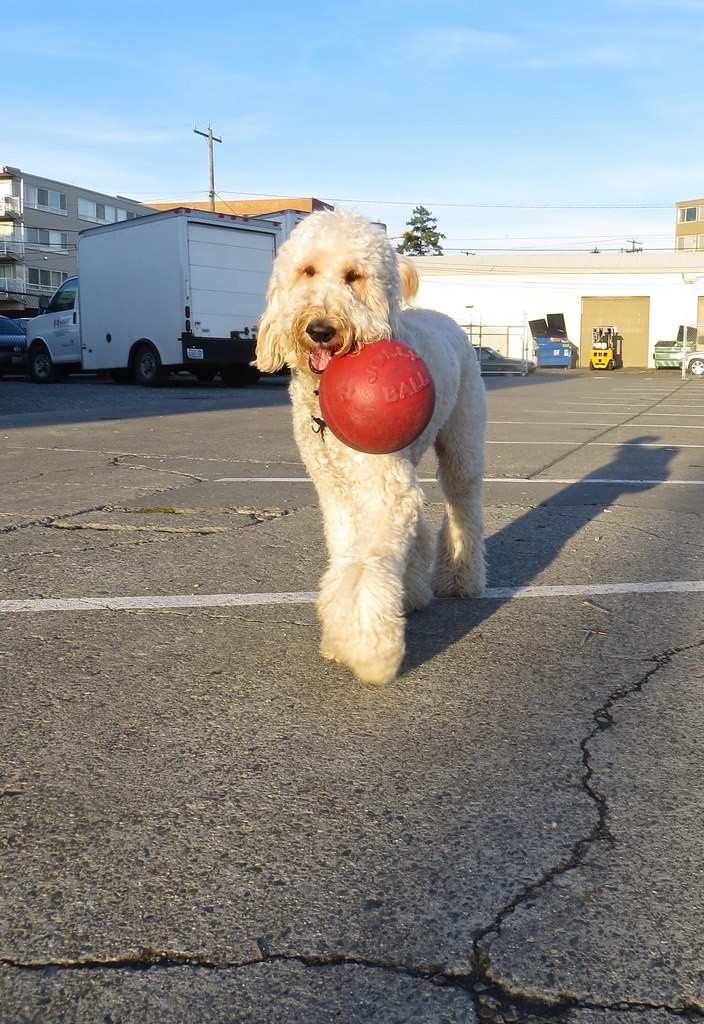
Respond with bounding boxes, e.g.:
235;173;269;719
473;346;537;377
685;350;704;376
0;316;35;378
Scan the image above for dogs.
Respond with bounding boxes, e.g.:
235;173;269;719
252;211;492;684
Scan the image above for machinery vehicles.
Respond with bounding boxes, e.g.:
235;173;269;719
588;327;616;371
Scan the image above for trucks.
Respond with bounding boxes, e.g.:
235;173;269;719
26;207;285;388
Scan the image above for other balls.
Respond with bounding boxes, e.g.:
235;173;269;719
319;340;435;456
